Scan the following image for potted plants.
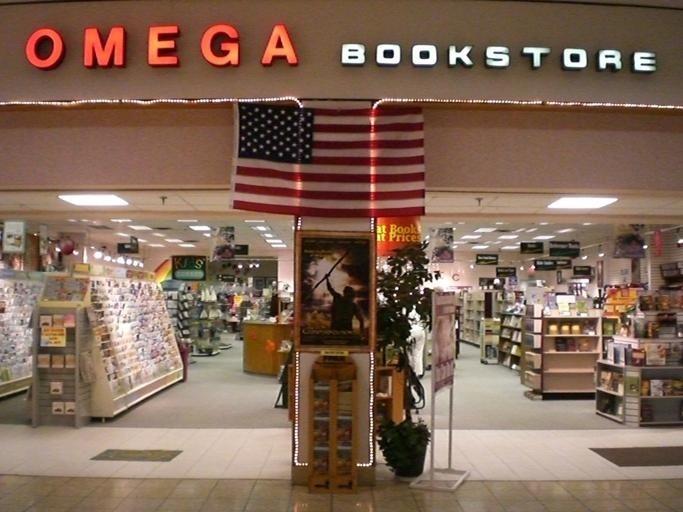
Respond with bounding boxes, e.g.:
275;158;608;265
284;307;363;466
374;239;432;477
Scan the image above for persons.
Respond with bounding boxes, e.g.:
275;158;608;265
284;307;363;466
622;232;644;257
214;245;234;261
326;273;365;332
436;246;453;261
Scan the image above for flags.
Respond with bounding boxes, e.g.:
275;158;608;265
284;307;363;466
229;103;426;220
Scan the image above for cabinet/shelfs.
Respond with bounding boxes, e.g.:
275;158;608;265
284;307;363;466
0;268;203;422
453;287;683;428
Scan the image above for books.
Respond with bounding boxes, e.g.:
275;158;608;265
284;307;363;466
312;380;352;475
0;279;191;416
485;284;683;422
